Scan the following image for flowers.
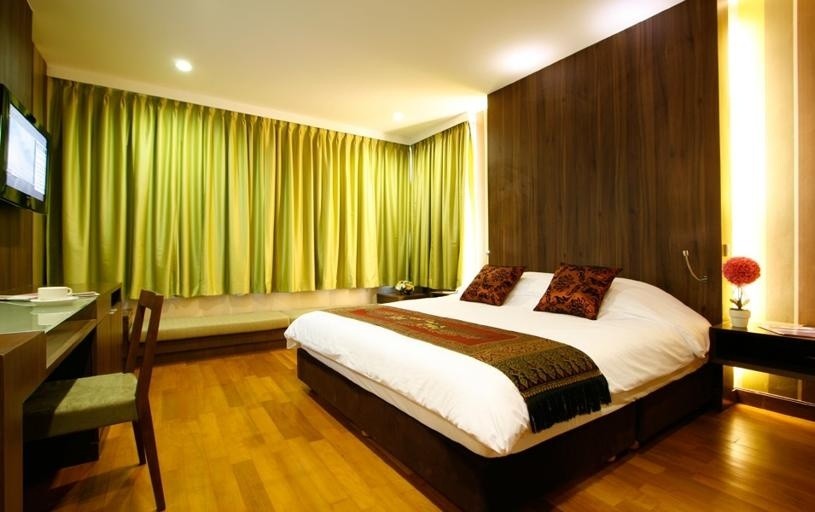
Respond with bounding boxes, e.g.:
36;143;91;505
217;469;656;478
395;280;416;293
722;256;761;310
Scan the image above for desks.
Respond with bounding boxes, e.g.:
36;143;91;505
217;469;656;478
2;281;123;511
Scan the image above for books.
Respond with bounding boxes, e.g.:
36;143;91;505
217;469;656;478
0;290;40;300
758;324;815;338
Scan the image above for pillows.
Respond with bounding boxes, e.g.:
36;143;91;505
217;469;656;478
461;263;526;308
533;261;624;320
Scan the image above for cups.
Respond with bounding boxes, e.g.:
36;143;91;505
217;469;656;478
38;286;72;299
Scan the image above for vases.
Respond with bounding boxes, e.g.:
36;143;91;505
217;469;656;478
728;308;750;330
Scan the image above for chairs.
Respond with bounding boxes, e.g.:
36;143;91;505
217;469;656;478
19;288;166;512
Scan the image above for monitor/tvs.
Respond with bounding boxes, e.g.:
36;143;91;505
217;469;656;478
0;83;53;215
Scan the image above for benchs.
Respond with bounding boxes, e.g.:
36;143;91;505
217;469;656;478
122;287;378;372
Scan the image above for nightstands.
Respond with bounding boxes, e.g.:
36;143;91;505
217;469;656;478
376;290;428;305
709;319;814;423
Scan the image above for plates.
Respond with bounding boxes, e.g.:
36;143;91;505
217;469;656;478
29;296;78;306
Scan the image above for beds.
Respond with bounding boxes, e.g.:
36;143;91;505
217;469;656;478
283;270;722;511
